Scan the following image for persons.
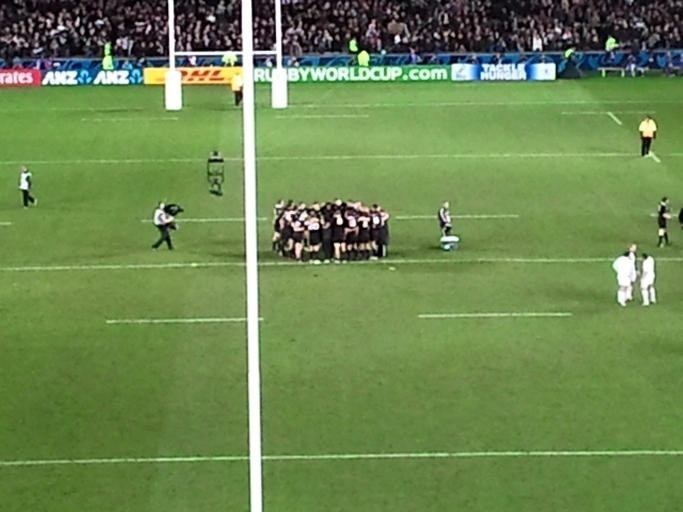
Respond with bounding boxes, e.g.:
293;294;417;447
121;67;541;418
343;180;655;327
438;201;452;250
613;244;638;306
1;0;683;78
640;253;656;306
19;165;37;209
231;73;243;106
272;198;389;265
639;115;657;156
207;151;225;196
151;202;176;251
658;197;672;248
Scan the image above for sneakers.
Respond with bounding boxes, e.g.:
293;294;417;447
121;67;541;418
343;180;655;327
273;248;387;265
